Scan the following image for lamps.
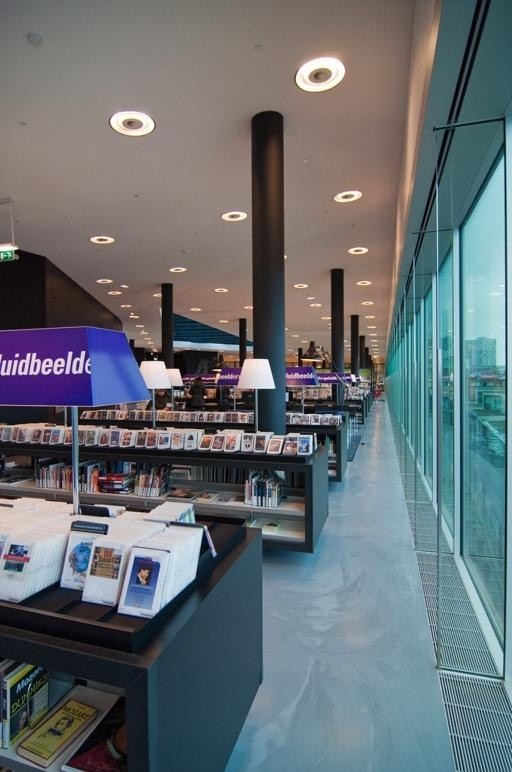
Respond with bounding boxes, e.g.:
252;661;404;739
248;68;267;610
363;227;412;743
298;341;323;363
0;197;19;252
236;358;276;433
135;358;184;431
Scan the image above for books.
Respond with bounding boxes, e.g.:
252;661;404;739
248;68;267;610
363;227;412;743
0;497;217;620
75;409;342;457
1;421;73;445
286;383;370;401
0;454;170;514
0;656;128;771
173;461;306;535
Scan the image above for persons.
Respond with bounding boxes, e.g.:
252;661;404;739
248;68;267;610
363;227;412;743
189;377;208;411
145;389;178;411
216;386;232;412
